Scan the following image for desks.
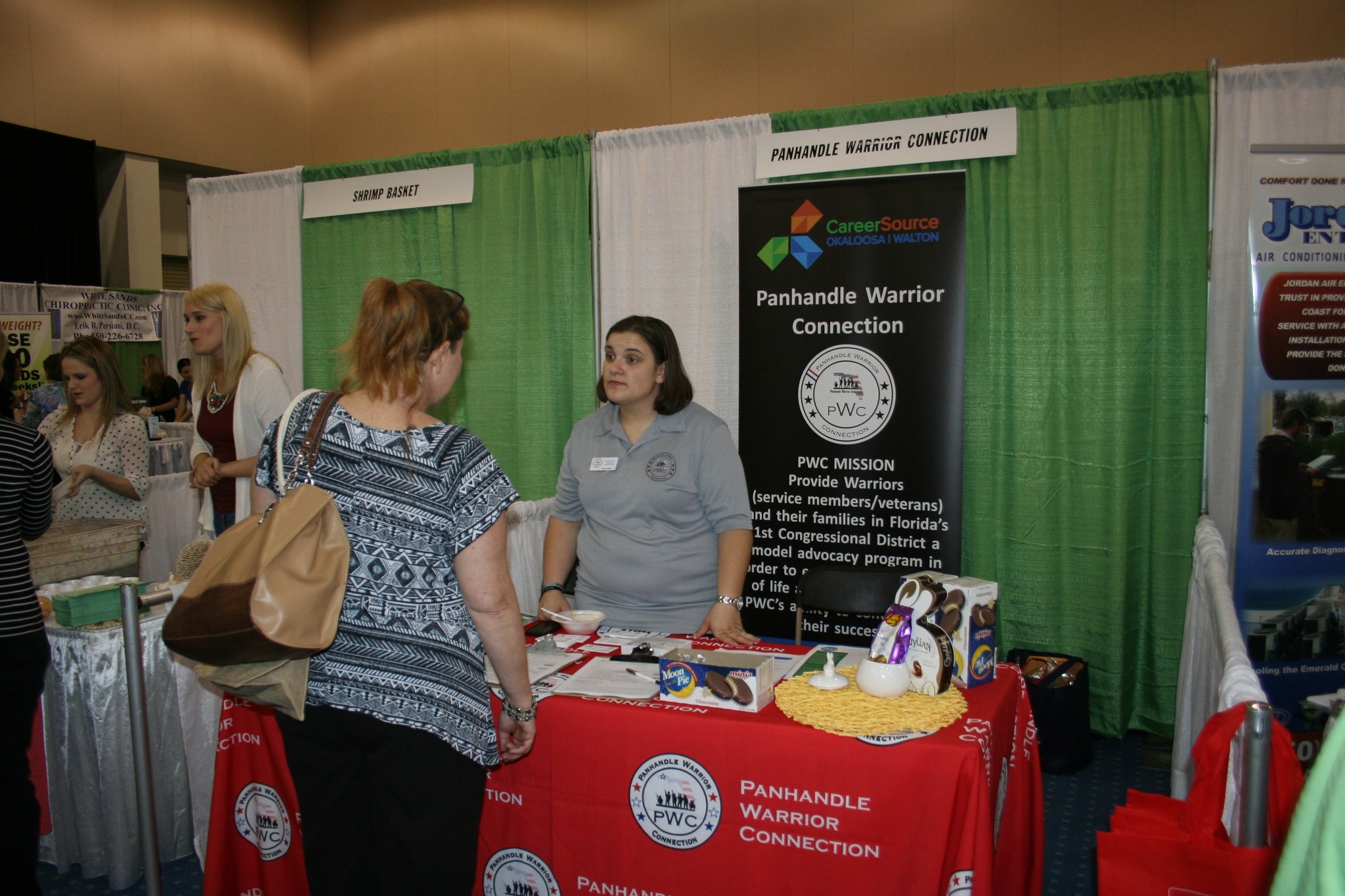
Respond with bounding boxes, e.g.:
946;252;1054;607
201;621;1045;896
25;602;197;891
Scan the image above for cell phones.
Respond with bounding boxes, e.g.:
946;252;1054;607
524;619;561;636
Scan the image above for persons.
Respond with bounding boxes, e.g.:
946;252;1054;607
139;354;194;422
1258;408;1320;544
182;283;293;538
37;335;151;541
252;276;539;896
0;330;67;896
538;314;760;647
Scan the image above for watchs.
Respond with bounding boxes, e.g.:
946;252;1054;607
715;595;743;611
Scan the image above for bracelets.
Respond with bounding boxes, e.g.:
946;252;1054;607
500;697;537;722
539;586;562;602
541;582;564;592
152;407;155;412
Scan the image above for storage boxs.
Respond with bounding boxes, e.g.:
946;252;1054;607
22;519;146;587
901;570;1000;688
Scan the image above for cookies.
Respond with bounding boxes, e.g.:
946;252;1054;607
705;671;753;705
939;590;995;633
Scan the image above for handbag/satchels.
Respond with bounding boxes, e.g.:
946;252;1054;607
161;390;350;665
194;655;310;721
1005;647;1089;774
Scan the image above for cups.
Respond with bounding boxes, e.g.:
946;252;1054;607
857;656;910;699
36;575;138;602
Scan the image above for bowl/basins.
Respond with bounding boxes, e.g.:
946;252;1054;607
550;609;607;635
52;473;75;501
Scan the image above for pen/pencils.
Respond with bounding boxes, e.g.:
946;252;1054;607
625;667;661;685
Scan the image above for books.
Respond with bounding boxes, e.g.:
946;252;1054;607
1306;455;1336;469
